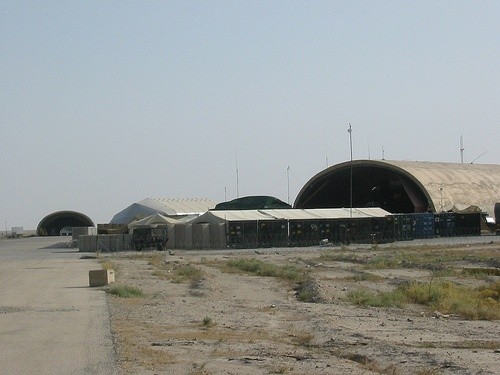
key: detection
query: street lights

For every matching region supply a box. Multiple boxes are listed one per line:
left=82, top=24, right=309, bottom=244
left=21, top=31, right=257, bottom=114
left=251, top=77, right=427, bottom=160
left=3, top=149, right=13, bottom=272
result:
left=347, top=123, right=354, bottom=218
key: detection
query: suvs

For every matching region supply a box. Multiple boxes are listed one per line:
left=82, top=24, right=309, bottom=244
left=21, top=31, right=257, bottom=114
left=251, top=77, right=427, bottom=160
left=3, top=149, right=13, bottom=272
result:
left=132, top=228, right=168, bottom=251
